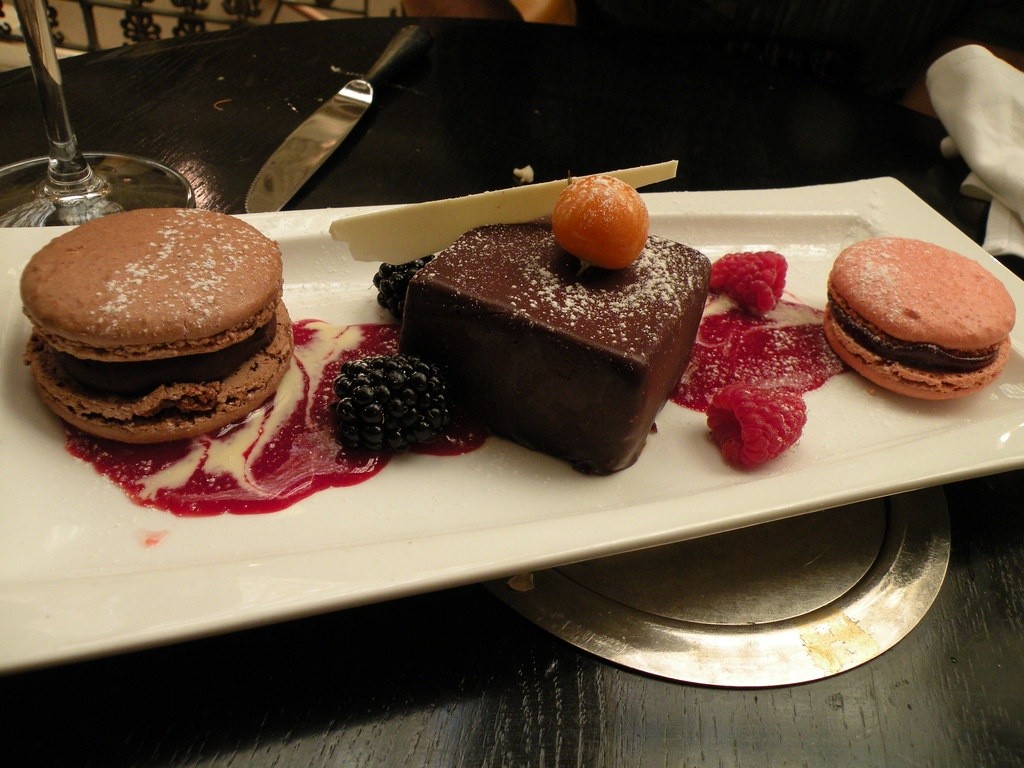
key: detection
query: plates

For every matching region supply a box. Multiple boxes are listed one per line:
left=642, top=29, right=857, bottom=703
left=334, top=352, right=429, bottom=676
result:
left=0, top=176, right=1024, bottom=677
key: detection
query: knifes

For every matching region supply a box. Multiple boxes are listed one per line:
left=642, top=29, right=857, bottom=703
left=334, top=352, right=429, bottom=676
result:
left=241, top=20, right=441, bottom=215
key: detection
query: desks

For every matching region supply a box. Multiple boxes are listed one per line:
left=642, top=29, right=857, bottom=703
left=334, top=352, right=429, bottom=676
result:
left=0, top=21, right=1024, bottom=767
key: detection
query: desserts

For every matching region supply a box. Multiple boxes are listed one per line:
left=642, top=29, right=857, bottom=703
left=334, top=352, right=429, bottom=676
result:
left=326, top=160, right=715, bottom=474
left=19, top=202, right=297, bottom=442
left=822, top=236, right=1016, bottom=398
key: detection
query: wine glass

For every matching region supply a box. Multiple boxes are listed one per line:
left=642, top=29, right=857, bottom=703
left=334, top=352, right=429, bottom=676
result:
left=0, top=0, right=197, bottom=229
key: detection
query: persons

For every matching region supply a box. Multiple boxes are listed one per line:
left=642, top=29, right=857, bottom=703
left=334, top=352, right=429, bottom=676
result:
left=404, top=1, right=1023, bottom=120
